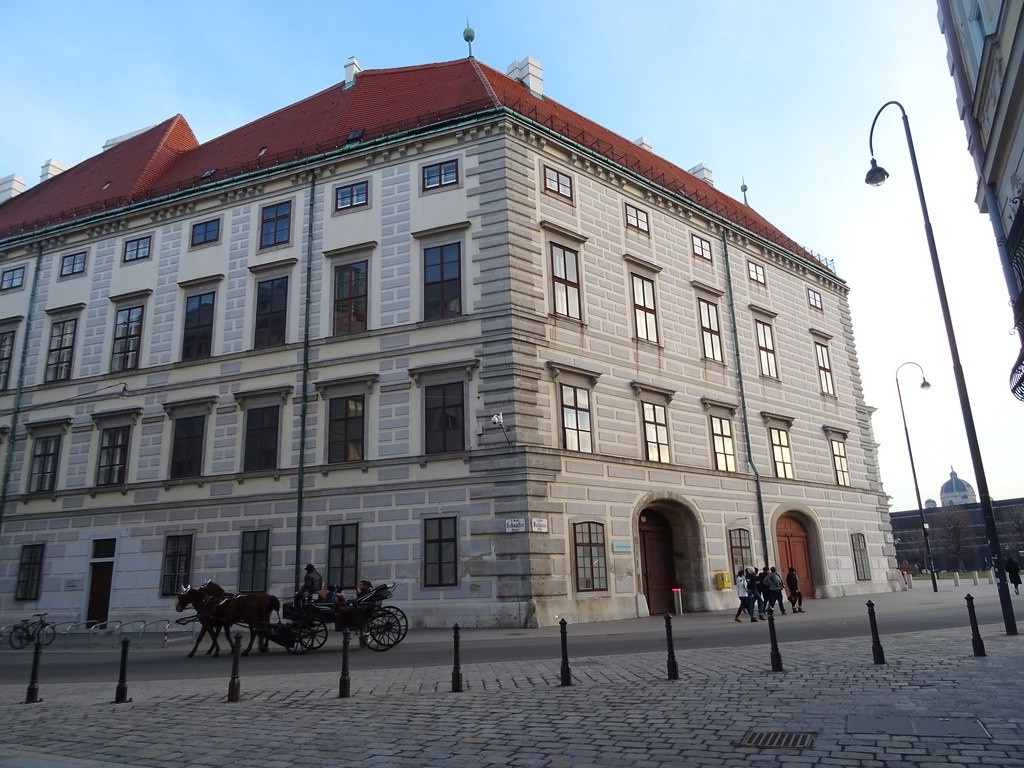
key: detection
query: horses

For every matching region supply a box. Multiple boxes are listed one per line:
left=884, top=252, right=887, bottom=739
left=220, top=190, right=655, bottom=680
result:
left=175, top=583, right=271, bottom=659
left=198, top=578, right=281, bottom=656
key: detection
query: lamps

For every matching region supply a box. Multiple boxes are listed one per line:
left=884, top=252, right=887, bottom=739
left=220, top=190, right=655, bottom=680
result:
left=490, top=411, right=511, bottom=447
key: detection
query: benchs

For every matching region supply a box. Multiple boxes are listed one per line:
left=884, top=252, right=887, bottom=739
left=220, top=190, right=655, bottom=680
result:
left=333, top=581, right=397, bottom=631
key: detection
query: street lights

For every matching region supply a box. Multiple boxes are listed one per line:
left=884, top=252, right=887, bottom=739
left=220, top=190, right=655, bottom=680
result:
left=896, top=362, right=942, bottom=593
left=865, top=101, right=1018, bottom=636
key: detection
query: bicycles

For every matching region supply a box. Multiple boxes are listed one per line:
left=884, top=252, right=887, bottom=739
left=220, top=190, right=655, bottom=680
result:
left=9, top=612, right=57, bottom=650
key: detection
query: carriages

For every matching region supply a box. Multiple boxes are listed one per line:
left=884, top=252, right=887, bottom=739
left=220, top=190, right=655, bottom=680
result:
left=173, top=563, right=409, bottom=655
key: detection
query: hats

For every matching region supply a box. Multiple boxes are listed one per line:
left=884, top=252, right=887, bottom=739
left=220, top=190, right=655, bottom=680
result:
left=304, top=564, right=314, bottom=571
left=747, top=566, right=756, bottom=574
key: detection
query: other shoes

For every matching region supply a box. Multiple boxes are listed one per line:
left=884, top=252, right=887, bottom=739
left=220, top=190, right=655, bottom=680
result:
left=782, top=611, right=787, bottom=615
left=735, top=617, right=741, bottom=622
left=759, top=615, right=765, bottom=620
left=798, top=606, right=805, bottom=612
left=751, top=617, right=758, bottom=621
left=793, top=608, right=799, bottom=613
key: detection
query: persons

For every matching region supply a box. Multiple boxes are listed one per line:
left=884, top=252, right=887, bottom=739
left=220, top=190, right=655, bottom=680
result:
left=295, top=564, right=373, bottom=612
left=1006, top=556, right=1022, bottom=594
left=734, top=566, right=806, bottom=622
left=915, top=563, right=923, bottom=575
